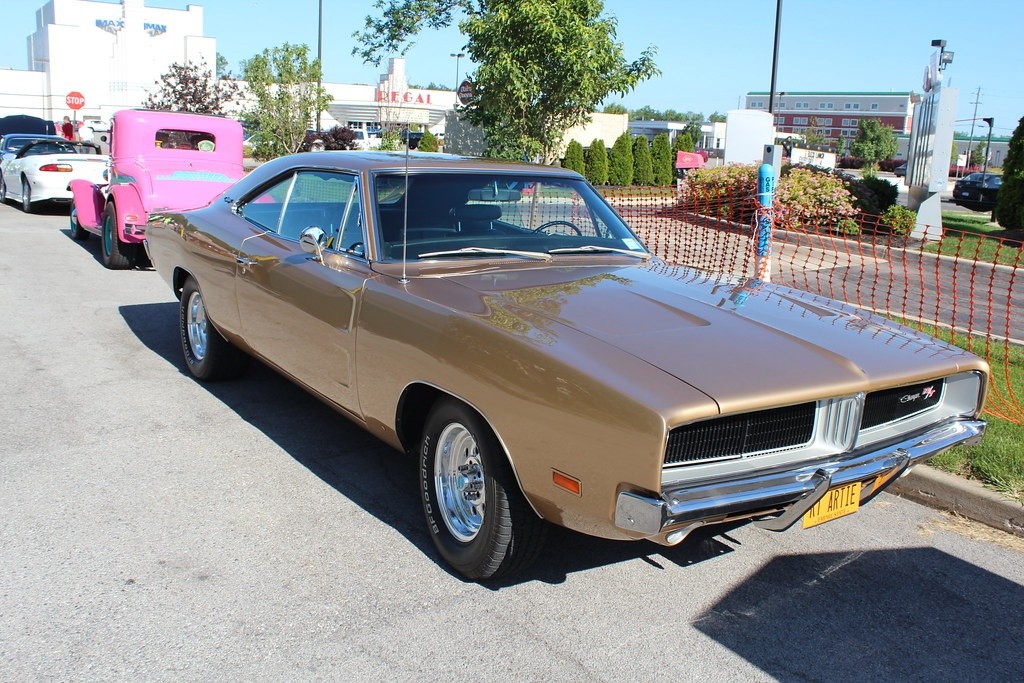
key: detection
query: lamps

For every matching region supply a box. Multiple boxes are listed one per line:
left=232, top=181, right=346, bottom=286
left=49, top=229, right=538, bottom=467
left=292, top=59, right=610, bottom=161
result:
left=931, top=39, right=954, bottom=70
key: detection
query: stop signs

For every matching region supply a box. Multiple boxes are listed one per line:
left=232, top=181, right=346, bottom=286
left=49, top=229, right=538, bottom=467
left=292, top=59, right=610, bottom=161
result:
left=66, top=92, right=85, bottom=109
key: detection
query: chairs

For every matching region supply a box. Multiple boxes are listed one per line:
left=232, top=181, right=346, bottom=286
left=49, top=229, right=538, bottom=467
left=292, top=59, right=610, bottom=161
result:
left=450, top=203, right=503, bottom=237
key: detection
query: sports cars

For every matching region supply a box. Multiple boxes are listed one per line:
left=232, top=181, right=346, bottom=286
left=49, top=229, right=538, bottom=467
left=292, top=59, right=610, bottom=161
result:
left=0, top=140, right=111, bottom=213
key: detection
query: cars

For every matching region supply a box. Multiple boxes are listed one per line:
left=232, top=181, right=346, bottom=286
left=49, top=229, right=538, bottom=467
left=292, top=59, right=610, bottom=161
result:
left=0, top=134, right=77, bottom=164
left=142, top=149, right=991, bottom=581
left=953, top=173, right=1003, bottom=203
left=55, top=119, right=111, bottom=132
left=67, top=108, right=276, bottom=271
left=894, top=163, right=907, bottom=177
left=695, top=146, right=708, bottom=163
left=242, top=128, right=445, bottom=150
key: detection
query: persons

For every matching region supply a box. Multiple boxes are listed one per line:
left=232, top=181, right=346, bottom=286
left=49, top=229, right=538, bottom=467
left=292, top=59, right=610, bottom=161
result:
left=77, top=122, right=94, bottom=143
left=62, top=115, right=73, bottom=140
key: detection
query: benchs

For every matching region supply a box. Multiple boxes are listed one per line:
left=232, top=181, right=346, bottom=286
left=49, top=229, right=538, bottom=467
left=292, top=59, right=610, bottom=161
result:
left=259, top=207, right=410, bottom=249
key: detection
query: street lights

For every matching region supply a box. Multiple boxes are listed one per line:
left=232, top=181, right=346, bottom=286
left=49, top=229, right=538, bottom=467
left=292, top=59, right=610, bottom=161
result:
left=931, top=39, right=947, bottom=72
left=450, top=53, right=465, bottom=103
left=776, top=92, right=785, bottom=145
